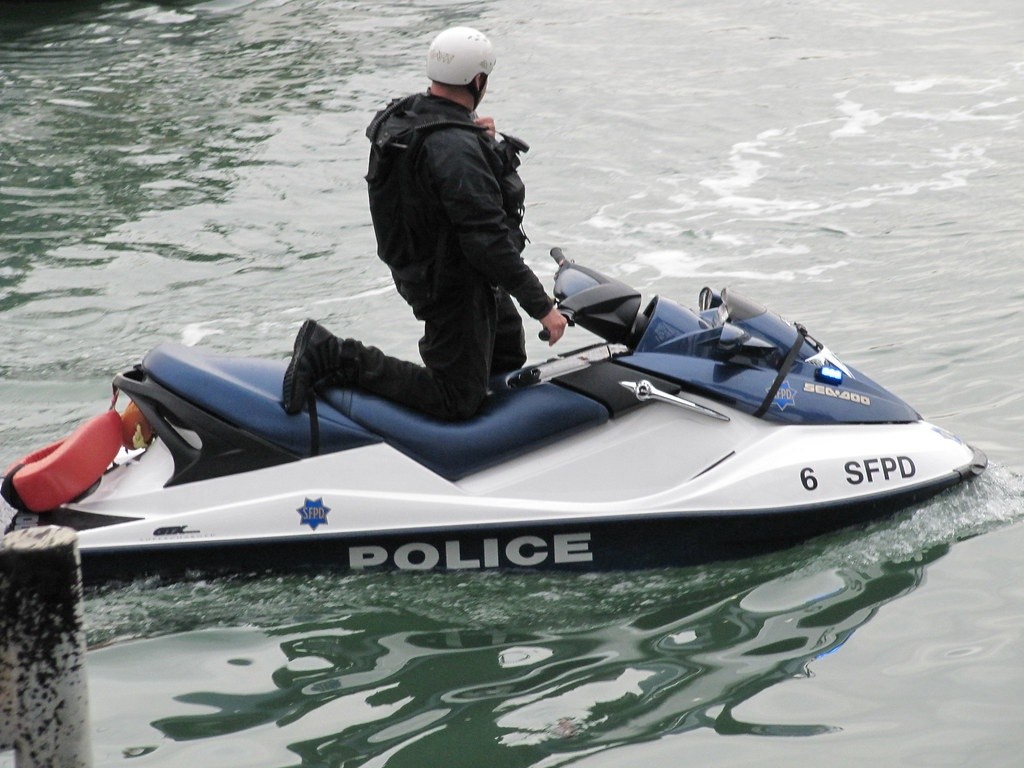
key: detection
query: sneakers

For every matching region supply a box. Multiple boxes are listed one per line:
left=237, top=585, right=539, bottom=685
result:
left=283, top=319, right=353, bottom=415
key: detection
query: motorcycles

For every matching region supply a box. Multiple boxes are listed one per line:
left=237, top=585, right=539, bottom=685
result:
left=0, top=245, right=990, bottom=584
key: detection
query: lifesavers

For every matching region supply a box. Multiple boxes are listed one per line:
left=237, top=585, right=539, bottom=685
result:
left=1, top=410, right=123, bottom=513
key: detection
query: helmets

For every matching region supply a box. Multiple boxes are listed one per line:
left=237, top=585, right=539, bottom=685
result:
left=425, top=26, right=496, bottom=86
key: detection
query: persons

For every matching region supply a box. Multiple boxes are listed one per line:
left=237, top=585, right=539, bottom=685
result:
left=283, top=23, right=568, bottom=422
